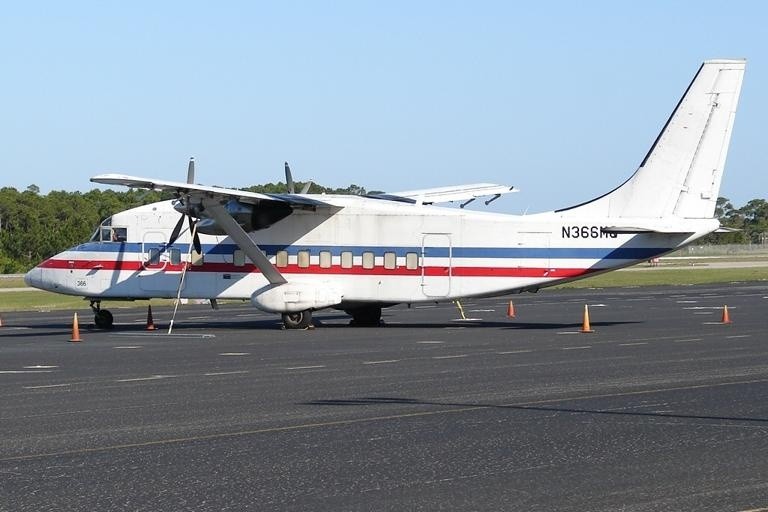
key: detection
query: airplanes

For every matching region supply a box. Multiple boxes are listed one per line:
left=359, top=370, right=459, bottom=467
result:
left=22, top=59, right=747, bottom=329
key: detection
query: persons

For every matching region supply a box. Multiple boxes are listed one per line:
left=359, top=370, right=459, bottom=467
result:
left=108, top=229, right=127, bottom=242
left=648, top=257, right=659, bottom=268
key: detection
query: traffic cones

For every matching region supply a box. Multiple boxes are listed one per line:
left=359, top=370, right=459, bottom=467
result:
left=64, top=312, right=83, bottom=343
left=578, top=303, right=596, bottom=332
left=142, top=304, right=159, bottom=331
left=505, top=298, right=516, bottom=319
left=719, top=304, right=735, bottom=323
